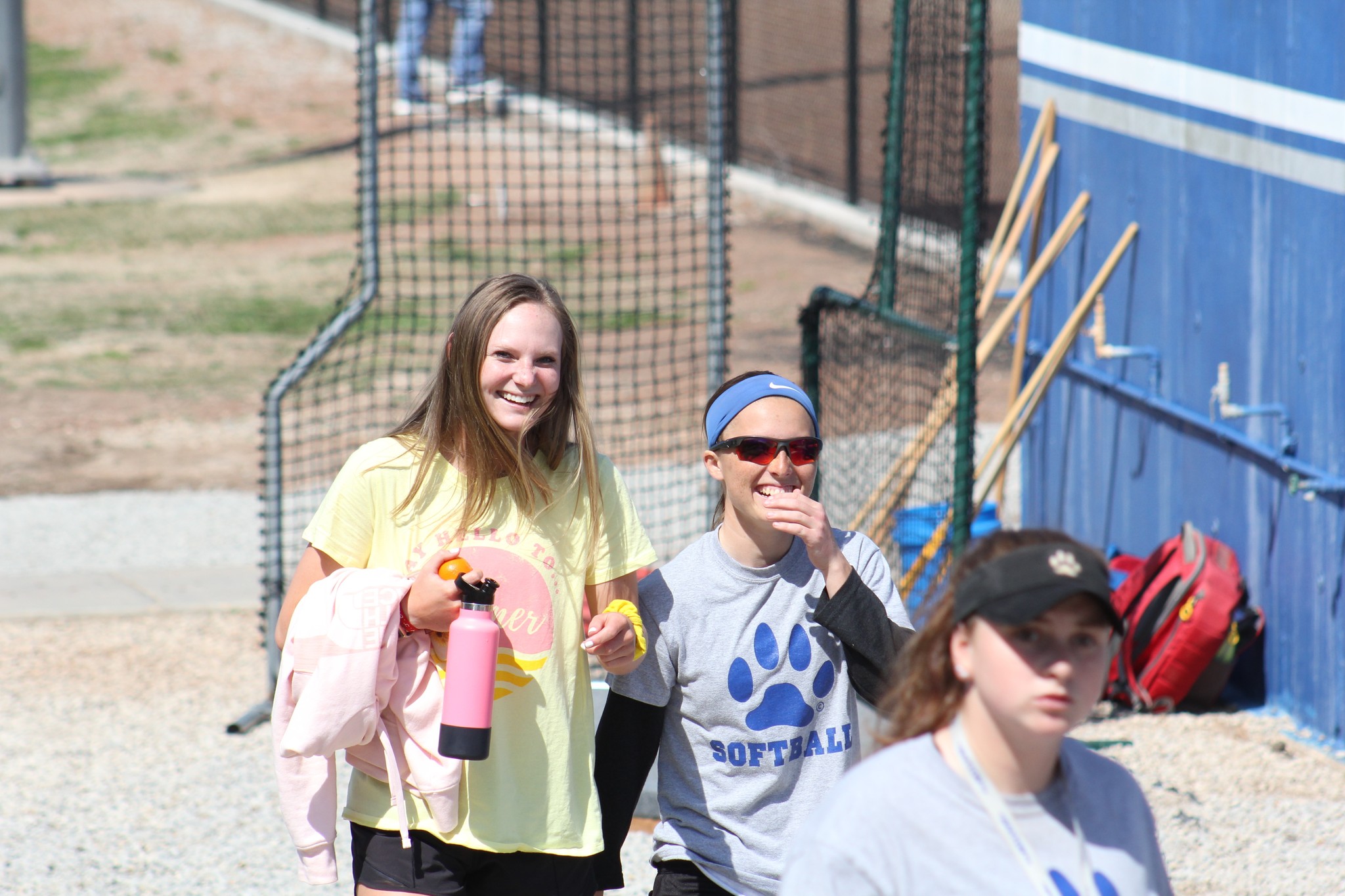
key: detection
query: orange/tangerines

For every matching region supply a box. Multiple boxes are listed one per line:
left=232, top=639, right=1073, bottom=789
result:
left=438, top=556, right=473, bottom=580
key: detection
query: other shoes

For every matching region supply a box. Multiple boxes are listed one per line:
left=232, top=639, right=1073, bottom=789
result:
left=454, top=79, right=503, bottom=93
left=397, top=100, right=445, bottom=114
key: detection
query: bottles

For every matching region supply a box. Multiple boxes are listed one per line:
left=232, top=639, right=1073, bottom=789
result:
left=437, top=572, right=499, bottom=760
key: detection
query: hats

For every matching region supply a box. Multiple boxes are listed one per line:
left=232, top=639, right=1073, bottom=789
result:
left=951, top=542, right=1125, bottom=637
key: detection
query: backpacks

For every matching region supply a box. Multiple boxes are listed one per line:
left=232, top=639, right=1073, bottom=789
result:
left=1104, top=521, right=1265, bottom=715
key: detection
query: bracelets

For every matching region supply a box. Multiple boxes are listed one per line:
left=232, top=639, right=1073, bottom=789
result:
left=601, top=600, right=647, bottom=661
left=400, top=602, right=419, bottom=632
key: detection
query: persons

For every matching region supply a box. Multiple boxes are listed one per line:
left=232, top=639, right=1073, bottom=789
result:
left=274, top=273, right=658, bottom=896
left=774, top=529, right=1176, bottom=895
left=593, top=370, right=917, bottom=896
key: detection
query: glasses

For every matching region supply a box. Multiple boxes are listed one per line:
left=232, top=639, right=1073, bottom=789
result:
left=710, top=436, right=823, bottom=466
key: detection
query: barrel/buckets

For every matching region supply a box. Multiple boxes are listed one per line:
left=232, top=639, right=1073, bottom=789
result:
left=894, top=502, right=999, bottom=636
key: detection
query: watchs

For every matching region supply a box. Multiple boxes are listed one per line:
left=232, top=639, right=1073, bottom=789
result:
left=398, top=624, right=413, bottom=640
